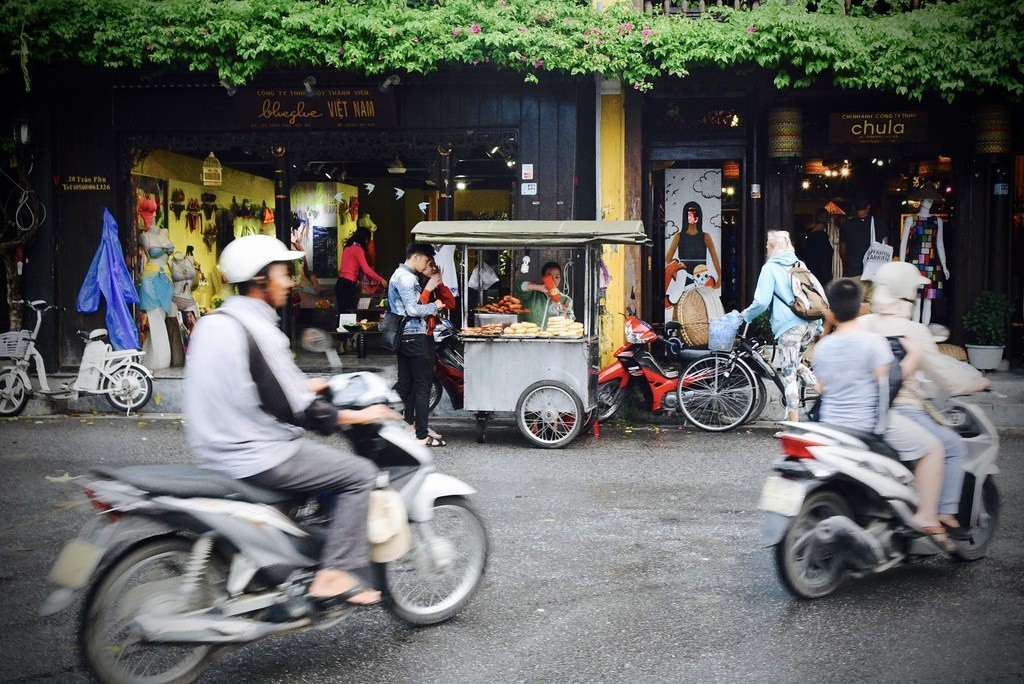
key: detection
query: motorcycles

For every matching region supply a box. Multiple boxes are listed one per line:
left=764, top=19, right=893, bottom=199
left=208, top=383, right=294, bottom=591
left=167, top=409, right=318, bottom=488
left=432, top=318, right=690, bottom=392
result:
left=596, top=307, right=767, bottom=432
left=39, top=332, right=489, bottom=684
left=409, top=220, right=651, bottom=450
left=753, top=324, right=1001, bottom=602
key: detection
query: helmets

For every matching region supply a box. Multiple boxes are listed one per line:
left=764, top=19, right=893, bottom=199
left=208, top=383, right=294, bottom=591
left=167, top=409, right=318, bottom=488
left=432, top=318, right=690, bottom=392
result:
left=433, top=318, right=458, bottom=343
left=871, top=261, right=932, bottom=300
left=217, top=233, right=306, bottom=283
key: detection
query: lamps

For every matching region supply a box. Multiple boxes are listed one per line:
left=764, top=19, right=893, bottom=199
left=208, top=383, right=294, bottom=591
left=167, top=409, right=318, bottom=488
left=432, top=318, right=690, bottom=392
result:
left=303, top=163, right=346, bottom=182
left=387, top=154, right=407, bottom=173
left=380, top=74, right=401, bottom=89
left=218, top=78, right=238, bottom=96
left=303, top=76, right=316, bottom=92
left=485, top=144, right=516, bottom=169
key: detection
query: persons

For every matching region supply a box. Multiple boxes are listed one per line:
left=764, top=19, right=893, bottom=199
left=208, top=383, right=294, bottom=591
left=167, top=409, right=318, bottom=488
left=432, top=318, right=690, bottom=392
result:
left=337, top=227, right=388, bottom=312
left=138, top=226, right=198, bottom=315
left=385, top=242, right=576, bottom=448
left=665, top=201, right=722, bottom=290
left=809, top=195, right=989, bottom=554
left=186, top=235, right=405, bottom=613
left=899, top=184, right=949, bottom=326
left=741, top=229, right=831, bottom=439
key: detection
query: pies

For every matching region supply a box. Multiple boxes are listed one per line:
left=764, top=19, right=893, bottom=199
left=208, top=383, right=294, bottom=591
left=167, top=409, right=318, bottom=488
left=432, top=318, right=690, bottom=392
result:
left=461, top=322, right=508, bottom=335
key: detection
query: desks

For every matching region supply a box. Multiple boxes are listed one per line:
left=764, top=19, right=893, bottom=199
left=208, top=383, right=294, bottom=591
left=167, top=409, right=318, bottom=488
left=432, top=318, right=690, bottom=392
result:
left=329, top=331, right=385, bottom=359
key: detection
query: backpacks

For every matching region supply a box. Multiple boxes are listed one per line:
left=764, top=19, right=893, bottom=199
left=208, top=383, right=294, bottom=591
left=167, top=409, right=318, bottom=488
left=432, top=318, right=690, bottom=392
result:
left=774, top=260, right=829, bottom=321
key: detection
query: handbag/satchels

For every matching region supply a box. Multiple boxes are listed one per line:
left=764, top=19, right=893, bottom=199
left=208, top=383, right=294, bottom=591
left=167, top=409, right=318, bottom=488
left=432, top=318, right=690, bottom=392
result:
left=859, top=215, right=894, bottom=282
left=378, top=312, right=412, bottom=353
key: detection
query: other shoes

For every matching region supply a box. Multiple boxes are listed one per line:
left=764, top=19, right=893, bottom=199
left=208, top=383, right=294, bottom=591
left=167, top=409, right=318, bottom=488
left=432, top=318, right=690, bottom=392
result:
left=939, top=519, right=972, bottom=539
left=773, top=431, right=783, bottom=438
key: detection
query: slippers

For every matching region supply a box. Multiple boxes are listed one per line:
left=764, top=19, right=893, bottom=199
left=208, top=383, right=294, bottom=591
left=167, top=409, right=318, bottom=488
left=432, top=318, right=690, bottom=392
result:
left=304, top=580, right=387, bottom=609
left=408, top=424, right=447, bottom=447
left=906, top=520, right=957, bottom=552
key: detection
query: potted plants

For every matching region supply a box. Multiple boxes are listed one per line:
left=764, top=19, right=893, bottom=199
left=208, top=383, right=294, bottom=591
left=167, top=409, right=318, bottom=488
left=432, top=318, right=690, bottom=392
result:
left=962, top=292, right=1016, bottom=369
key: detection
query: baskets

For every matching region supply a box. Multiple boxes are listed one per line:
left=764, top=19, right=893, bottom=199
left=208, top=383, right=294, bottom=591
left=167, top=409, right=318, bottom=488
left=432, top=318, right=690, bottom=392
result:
left=0, top=330, right=32, bottom=358
left=708, top=316, right=744, bottom=352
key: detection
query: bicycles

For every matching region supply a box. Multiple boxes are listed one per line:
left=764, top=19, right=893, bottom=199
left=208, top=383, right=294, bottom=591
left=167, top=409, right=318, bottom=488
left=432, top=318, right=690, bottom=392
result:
left=676, top=314, right=891, bottom=433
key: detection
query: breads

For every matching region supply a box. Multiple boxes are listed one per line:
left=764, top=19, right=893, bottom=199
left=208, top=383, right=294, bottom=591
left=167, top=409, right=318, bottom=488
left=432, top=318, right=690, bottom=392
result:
left=477, top=295, right=532, bottom=314
left=503, top=315, right=584, bottom=338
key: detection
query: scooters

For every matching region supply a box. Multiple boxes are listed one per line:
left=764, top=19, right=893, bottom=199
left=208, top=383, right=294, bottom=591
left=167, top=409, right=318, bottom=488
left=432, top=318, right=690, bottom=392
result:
left=0, top=297, right=156, bottom=416
